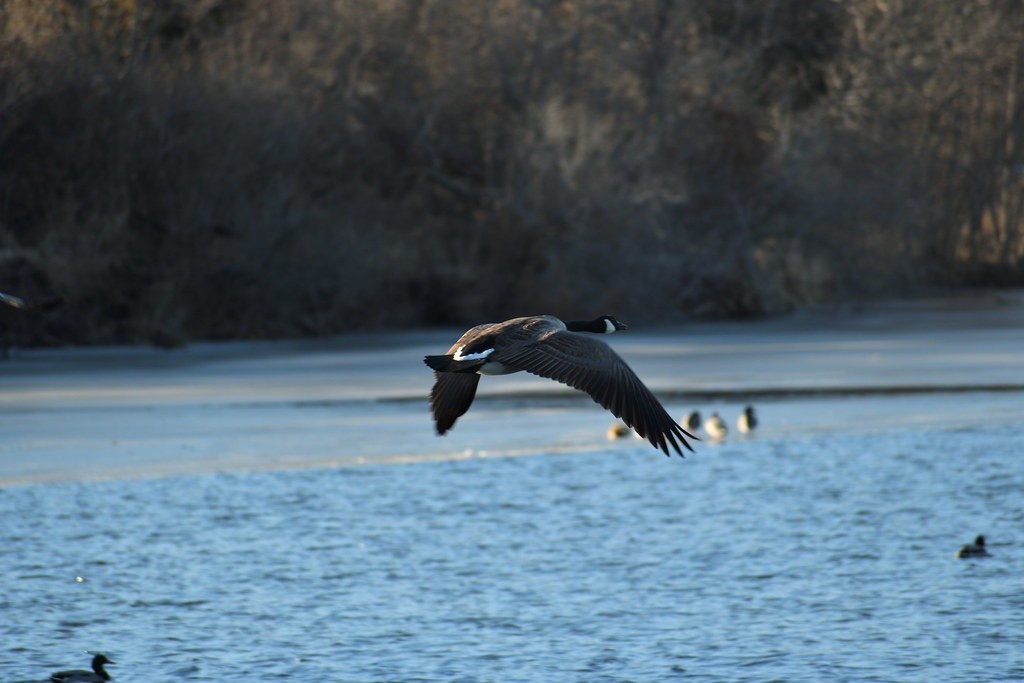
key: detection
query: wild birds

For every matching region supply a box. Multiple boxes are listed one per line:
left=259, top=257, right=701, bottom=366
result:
left=958, top=532, right=994, bottom=561
left=679, top=410, right=702, bottom=433
left=704, top=412, right=729, bottom=438
left=606, top=423, right=631, bottom=442
left=735, top=404, right=760, bottom=433
left=46, top=653, right=117, bottom=683
left=422, top=314, right=702, bottom=457
